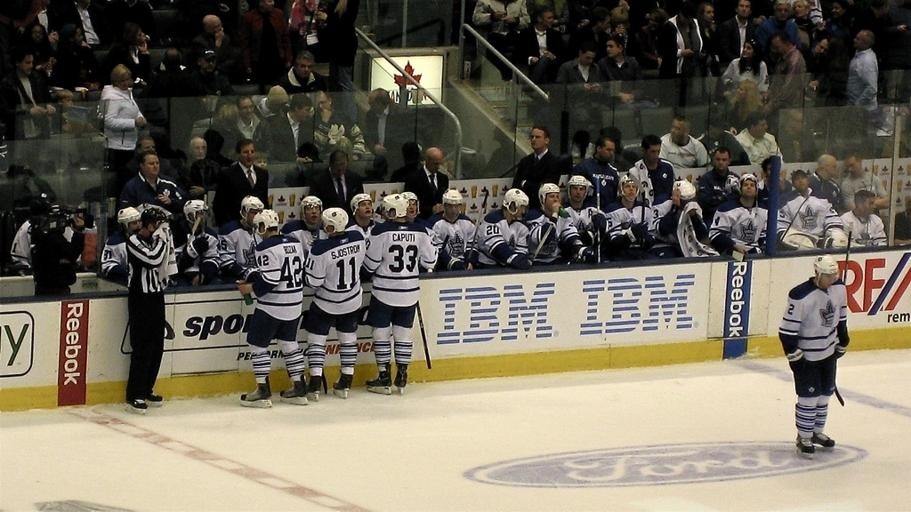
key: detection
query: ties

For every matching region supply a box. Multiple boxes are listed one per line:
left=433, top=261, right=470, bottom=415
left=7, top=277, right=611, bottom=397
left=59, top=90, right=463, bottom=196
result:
left=336, top=177, right=345, bottom=201
left=247, top=170, right=254, bottom=189
left=429, top=173, right=435, bottom=184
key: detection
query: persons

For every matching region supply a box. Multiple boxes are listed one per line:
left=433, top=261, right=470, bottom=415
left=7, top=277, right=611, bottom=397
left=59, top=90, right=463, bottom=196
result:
left=0, top=164, right=56, bottom=221
left=119, top=153, right=186, bottom=246
left=476, top=188, right=532, bottom=270
left=30, top=219, right=86, bottom=296
left=573, top=133, right=627, bottom=203
left=125, top=209, right=179, bottom=408
left=659, top=115, right=710, bottom=167
left=780, top=255, right=850, bottom=460
left=893, top=191, right=911, bottom=246
left=607, top=172, right=659, bottom=260
left=806, top=154, right=842, bottom=214
left=172, top=199, right=220, bottom=284
left=213, top=140, right=270, bottom=236
left=237, top=209, right=308, bottom=406
left=472, top=1, right=699, bottom=128
left=431, top=189, right=479, bottom=272
left=777, top=112, right=819, bottom=161
left=363, top=89, right=412, bottom=157
left=841, top=153, right=889, bottom=212
left=9, top=195, right=86, bottom=276
left=700, top=110, right=751, bottom=166
left=279, top=196, right=329, bottom=257
left=178, top=137, right=223, bottom=203
left=362, top=192, right=437, bottom=394
left=309, top=151, right=363, bottom=212
left=304, top=206, right=366, bottom=401
left=555, top=173, right=611, bottom=264
left=345, top=192, right=382, bottom=248
left=401, top=192, right=433, bottom=236
left=0, top=0, right=168, bottom=175
left=512, top=125, right=568, bottom=207
left=650, top=179, right=708, bottom=257
left=528, top=181, right=573, bottom=267
left=708, top=173, right=769, bottom=255
left=699, top=1, right=910, bottom=131
left=630, top=135, right=674, bottom=205
left=735, top=115, right=785, bottom=167
left=756, top=158, right=790, bottom=205
left=168, top=1, right=365, bottom=154
left=220, top=194, right=269, bottom=284
left=99, top=205, right=145, bottom=286
left=776, top=170, right=844, bottom=252
left=841, top=188, right=887, bottom=249
left=409, top=145, right=449, bottom=214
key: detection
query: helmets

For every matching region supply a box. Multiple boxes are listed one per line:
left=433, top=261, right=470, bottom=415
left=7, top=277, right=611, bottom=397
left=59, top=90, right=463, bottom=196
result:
left=740, top=174, right=758, bottom=192
left=814, top=255, right=838, bottom=275
left=117, top=207, right=141, bottom=229
left=617, top=174, right=640, bottom=197
left=538, top=183, right=560, bottom=204
left=401, top=192, right=421, bottom=215
left=568, top=176, right=588, bottom=200
left=350, top=194, right=371, bottom=216
left=502, top=188, right=529, bottom=215
left=183, top=200, right=209, bottom=224
left=383, top=194, right=409, bottom=219
left=240, top=195, right=264, bottom=219
left=253, top=209, right=279, bottom=235
left=141, top=208, right=166, bottom=226
left=300, top=196, right=323, bottom=218
left=443, top=190, right=463, bottom=205
left=321, top=208, right=349, bottom=234
left=673, top=179, right=696, bottom=200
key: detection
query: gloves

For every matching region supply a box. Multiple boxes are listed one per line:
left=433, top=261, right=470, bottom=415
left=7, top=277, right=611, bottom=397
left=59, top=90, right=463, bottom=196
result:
left=787, top=349, right=807, bottom=374
left=832, top=345, right=846, bottom=359
left=725, top=174, right=740, bottom=192
left=578, top=246, right=594, bottom=262
left=193, top=236, right=209, bottom=254
left=581, top=230, right=597, bottom=246
left=627, top=221, right=649, bottom=243
left=507, top=253, right=533, bottom=269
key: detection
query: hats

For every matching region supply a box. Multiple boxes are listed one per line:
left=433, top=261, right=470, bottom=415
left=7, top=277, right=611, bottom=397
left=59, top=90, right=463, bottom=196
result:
left=201, top=48, right=216, bottom=59
left=268, top=86, right=288, bottom=104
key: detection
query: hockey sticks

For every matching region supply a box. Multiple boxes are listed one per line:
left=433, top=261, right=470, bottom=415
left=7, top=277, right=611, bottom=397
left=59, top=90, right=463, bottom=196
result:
left=779, top=187, right=813, bottom=244
left=835, top=231, right=852, bottom=406
left=627, top=173, right=646, bottom=259
left=467, top=186, right=488, bottom=269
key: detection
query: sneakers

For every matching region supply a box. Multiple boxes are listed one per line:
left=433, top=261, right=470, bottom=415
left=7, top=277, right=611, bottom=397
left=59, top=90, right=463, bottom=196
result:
left=306, top=376, right=321, bottom=392
left=394, top=370, right=407, bottom=387
left=130, top=398, right=147, bottom=409
left=241, top=383, right=270, bottom=401
left=333, top=373, right=352, bottom=389
left=812, top=431, right=835, bottom=446
left=367, top=372, right=391, bottom=386
left=280, top=381, right=305, bottom=398
left=148, top=394, right=162, bottom=401
left=796, top=436, right=814, bottom=454
